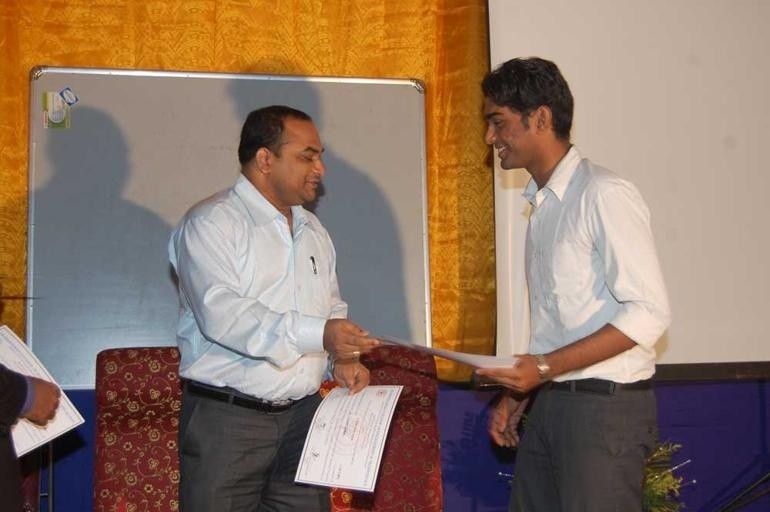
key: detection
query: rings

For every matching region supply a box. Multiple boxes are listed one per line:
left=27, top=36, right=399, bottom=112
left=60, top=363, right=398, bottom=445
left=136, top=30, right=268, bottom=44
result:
left=347, top=380, right=355, bottom=385
left=352, top=350, right=362, bottom=358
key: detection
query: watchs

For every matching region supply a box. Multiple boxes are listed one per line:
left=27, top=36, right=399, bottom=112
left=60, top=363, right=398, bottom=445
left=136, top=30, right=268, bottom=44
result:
left=535, top=353, right=550, bottom=385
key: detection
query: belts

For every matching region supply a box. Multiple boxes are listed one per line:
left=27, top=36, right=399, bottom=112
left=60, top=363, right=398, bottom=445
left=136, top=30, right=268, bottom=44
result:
left=180, top=381, right=307, bottom=413
left=539, top=376, right=656, bottom=397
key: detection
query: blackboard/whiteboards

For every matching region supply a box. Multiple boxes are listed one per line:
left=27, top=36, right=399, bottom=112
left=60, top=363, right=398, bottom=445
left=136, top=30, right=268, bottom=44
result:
left=24, top=66, right=433, bottom=388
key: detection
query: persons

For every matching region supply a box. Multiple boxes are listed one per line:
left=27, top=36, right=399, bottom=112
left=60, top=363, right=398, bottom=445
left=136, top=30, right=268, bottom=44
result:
left=0, top=362, right=60, bottom=510
left=480, top=56, right=671, bottom=510
left=166, top=106, right=378, bottom=512
